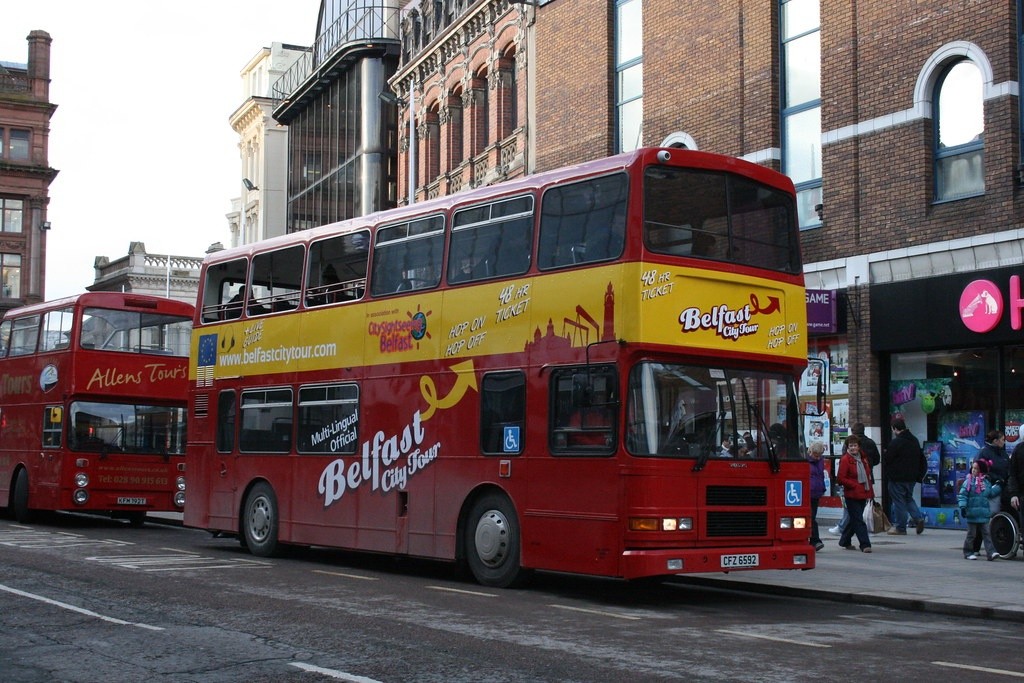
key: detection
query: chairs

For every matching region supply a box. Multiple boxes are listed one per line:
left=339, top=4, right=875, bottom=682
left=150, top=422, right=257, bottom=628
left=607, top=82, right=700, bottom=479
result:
left=396, top=279, right=412, bottom=291
left=355, top=282, right=366, bottom=298
left=484, top=256, right=491, bottom=277
left=271, top=297, right=290, bottom=312
left=568, top=243, right=585, bottom=263
left=324, top=288, right=338, bottom=303
left=219, top=303, right=228, bottom=320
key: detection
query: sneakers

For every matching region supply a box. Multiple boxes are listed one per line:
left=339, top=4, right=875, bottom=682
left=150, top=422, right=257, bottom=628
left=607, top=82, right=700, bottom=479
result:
left=829, top=525, right=841, bottom=536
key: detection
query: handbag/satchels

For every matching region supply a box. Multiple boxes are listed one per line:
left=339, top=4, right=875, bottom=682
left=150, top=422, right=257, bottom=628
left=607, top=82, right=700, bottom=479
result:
left=916, top=452, right=928, bottom=483
left=862, top=498, right=891, bottom=533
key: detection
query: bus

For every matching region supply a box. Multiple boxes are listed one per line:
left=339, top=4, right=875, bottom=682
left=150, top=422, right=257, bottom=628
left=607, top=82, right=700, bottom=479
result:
left=182, top=144, right=827, bottom=591
left=0, top=290, right=196, bottom=525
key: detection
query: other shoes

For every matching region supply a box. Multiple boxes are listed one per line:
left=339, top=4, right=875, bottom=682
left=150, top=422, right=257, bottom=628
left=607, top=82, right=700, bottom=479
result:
left=840, top=542, right=855, bottom=550
left=815, top=543, right=825, bottom=550
left=966, top=554, right=978, bottom=560
left=988, top=553, right=998, bottom=561
left=863, top=546, right=873, bottom=553
left=915, top=518, right=924, bottom=534
left=888, top=529, right=907, bottom=535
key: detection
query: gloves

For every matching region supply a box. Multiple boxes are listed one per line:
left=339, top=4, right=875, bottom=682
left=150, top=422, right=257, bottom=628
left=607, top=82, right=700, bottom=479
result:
left=960, top=508, right=966, bottom=517
left=996, top=479, right=1003, bottom=488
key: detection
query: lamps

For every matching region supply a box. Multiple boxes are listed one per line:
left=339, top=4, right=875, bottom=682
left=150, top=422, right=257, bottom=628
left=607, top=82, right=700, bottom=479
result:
left=243, top=177, right=257, bottom=191
left=40, top=221, right=52, bottom=231
left=377, top=91, right=408, bottom=109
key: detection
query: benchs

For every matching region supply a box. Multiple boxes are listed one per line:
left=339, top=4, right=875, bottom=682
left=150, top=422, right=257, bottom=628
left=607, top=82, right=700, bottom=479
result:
left=132, top=345, right=174, bottom=355
left=118, top=346, right=134, bottom=352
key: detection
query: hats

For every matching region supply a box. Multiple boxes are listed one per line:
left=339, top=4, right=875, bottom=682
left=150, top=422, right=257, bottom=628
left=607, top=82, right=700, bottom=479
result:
left=851, top=422, right=865, bottom=435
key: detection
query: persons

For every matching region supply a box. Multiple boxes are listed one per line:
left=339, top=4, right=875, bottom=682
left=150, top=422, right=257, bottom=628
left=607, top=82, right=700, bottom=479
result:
left=829, top=423, right=881, bottom=535
left=973, top=431, right=1020, bottom=529
left=318, top=261, right=347, bottom=303
left=569, top=384, right=634, bottom=446
left=468, top=237, right=530, bottom=279
left=226, top=286, right=267, bottom=319
left=882, top=418, right=924, bottom=534
left=1009, top=425, right=1024, bottom=554
left=958, top=458, right=1002, bottom=560
left=727, top=246, right=740, bottom=260
left=582, top=206, right=626, bottom=262
left=486, top=392, right=523, bottom=453
left=691, top=235, right=716, bottom=257
left=807, top=442, right=825, bottom=551
left=720, top=423, right=786, bottom=459
left=837, top=435, right=875, bottom=553
